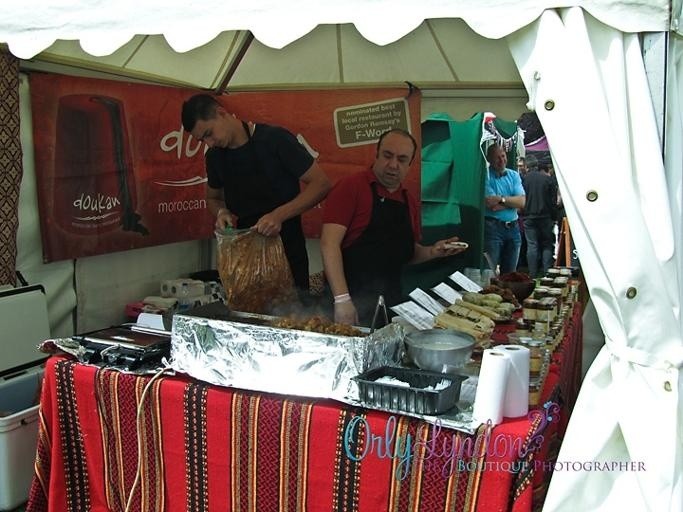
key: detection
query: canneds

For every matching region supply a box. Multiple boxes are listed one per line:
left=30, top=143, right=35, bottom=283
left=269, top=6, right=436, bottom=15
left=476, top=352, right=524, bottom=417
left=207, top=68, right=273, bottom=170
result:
left=515, top=268, right=578, bottom=410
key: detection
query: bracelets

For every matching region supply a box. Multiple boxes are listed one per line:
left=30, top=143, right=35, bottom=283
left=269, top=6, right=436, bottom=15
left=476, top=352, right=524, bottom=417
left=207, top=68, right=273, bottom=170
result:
left=499, top=197, right=506, bottom=205
left=333, top=293, right=351, bottom=304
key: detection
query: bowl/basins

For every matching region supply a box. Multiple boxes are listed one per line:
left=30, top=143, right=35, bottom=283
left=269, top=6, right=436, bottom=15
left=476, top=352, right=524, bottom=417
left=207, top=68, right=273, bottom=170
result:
left=403, top=329, right=476, bottom=374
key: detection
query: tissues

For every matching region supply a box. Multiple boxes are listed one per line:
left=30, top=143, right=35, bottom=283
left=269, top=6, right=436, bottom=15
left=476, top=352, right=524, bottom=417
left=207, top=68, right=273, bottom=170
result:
left=160, top=276, right=218, bottom=309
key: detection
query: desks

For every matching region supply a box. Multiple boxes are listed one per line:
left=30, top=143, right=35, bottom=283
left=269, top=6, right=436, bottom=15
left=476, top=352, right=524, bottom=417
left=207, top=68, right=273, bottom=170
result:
left=25, top=302, right=584, bottom=512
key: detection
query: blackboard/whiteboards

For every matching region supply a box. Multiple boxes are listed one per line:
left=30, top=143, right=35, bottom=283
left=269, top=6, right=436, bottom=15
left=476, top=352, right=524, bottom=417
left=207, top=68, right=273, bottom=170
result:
left=565, top=218, right=581, bottom=284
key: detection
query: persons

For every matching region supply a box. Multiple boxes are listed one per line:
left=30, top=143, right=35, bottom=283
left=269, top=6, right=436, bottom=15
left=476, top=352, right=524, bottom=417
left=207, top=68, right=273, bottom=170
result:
left=484, top=143, right=527, bottom=277
left=317, top=128, right=467, bottom=325
left=517, top=155, right=580, bottom=277
left=181, top=93, right=331, bottom=294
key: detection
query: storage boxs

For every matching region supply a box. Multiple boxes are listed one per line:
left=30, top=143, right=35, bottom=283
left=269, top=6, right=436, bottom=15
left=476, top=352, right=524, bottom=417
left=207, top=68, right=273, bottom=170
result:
left=0, top=271, right=52, bottom=512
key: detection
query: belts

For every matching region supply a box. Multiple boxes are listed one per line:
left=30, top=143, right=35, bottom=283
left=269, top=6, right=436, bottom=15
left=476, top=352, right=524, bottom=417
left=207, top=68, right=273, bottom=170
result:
left=486, top=217, right=519, bottom=229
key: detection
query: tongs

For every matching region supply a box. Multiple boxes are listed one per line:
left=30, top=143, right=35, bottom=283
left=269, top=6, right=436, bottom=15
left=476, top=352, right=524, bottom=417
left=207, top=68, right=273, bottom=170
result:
left=369, top=295, right=390, bottom=335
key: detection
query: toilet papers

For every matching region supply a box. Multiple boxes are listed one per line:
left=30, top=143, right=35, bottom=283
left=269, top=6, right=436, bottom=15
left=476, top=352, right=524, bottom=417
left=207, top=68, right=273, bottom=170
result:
left=472, top=348, right=507, bottom=427
left=495, top=344, right=531, bottom=419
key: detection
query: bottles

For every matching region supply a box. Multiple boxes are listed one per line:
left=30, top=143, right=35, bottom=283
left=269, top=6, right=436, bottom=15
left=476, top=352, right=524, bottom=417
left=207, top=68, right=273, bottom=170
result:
left=505, top=329, right=549, bottom=410
